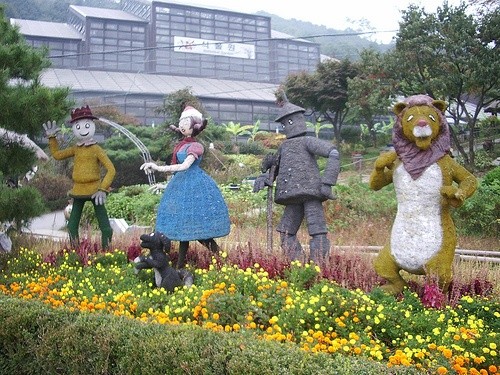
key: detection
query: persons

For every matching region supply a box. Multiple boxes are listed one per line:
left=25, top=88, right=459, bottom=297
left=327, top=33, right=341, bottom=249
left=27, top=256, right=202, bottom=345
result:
left=140, top=104, right=231, bottom=273
left=41, top=104, right=116, bottom=256
left=251, top=89, right=342, bottom=268
